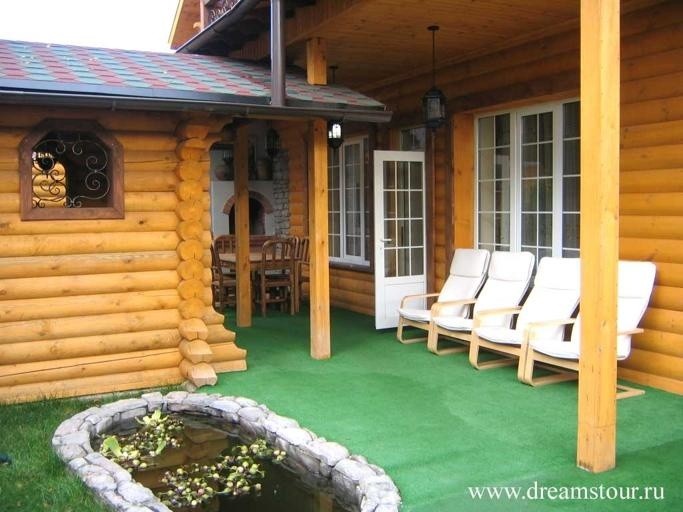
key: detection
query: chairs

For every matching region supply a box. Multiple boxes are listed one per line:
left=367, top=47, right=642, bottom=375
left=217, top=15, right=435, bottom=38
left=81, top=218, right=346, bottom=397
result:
left=473, top=255, right=584, bottom=387
left=524, top=258, right=658, bottom=401
left=209, top=233, right=309, bottom=320
left=432, top=249, right=536, bottom=372
left=389, top=246, right=490, bottom=359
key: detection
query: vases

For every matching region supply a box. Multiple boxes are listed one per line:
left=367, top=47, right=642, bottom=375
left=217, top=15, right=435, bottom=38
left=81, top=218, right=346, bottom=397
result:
left=256, top=162, right=272, bottom=180
left=214, top=165, right=233, bottom=181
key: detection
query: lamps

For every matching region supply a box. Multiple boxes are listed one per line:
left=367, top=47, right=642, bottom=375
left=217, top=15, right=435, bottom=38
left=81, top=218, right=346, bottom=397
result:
left=222, top=147, right=233, bottom=166
left=324, top=64, right=344, bottom=158
left=419, top=23, right=448, bottom=133
left=265, top=128, right=279, bottom=159
